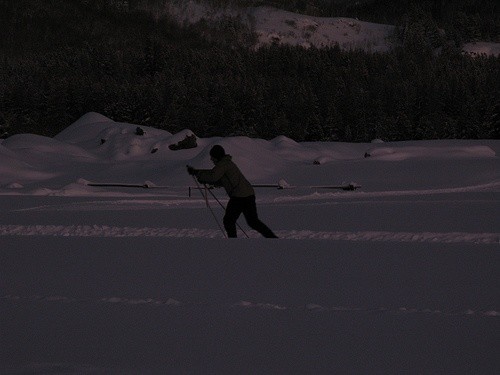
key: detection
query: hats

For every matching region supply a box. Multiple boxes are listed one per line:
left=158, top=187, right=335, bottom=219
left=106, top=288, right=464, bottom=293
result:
left=210, top=145, right=225, bottom=159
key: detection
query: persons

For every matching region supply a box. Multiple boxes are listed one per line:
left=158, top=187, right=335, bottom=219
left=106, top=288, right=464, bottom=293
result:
left=186, top=145, right=278, bottom=238
